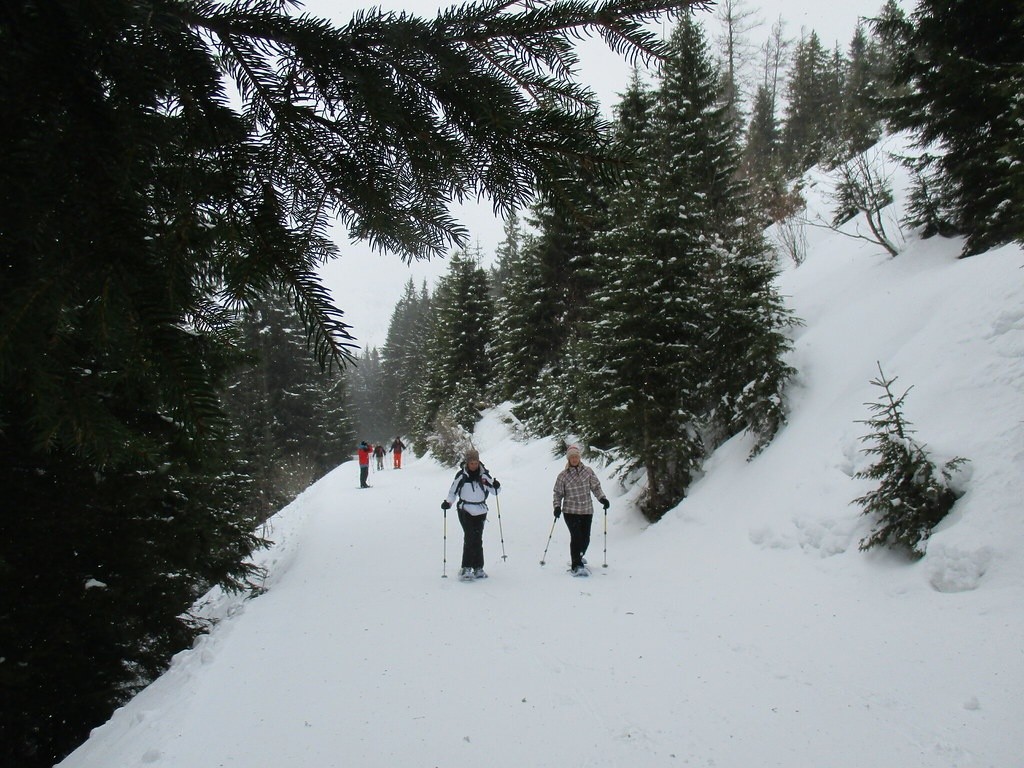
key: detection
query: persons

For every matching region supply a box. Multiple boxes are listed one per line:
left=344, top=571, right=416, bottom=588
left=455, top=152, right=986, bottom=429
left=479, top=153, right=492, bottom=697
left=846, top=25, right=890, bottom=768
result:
left=440, top=449, right=503, bottom=583
left=389, top=436, right=406, bottom=470
left=552, top=445, right=610, bottom=574
left=358, top=441, right=373, bottom=489
left=371, top=441, right=387, bottom=470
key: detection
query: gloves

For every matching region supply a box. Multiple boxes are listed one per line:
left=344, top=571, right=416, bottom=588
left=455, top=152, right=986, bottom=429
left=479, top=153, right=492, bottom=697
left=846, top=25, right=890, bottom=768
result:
left=493, top=478, right=500, bottom=488
left=600, top=498, right=609, bottom=509
left=441, top=500, right=450, bottom=509
left=554, top=507, right=561, bottom=518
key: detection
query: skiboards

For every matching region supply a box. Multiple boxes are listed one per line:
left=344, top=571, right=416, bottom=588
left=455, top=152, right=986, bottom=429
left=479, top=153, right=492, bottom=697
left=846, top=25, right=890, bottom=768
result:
left=458, top=569, right=490, bottom=583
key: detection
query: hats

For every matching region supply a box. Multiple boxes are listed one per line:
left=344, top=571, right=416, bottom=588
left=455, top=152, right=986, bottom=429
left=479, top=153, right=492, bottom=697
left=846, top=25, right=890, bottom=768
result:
left=465, top=450, right=479, bottom=463
left=361, top=441, right=367, bottom=446
left=376, top=441, right=380, bottom=444
left=566, top=445, right=580, bottom=459
left=396, top=436, right=400, bottom=440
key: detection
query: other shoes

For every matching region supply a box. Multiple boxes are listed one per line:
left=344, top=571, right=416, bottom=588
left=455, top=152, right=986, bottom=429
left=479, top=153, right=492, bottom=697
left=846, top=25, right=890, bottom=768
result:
left=581, top=558, right=587, bottom=564
left=462, top=567, right=472, bottom=578
left=475, top=568, right=486, bottom=578
left=571, top=566, right=584, bottom=574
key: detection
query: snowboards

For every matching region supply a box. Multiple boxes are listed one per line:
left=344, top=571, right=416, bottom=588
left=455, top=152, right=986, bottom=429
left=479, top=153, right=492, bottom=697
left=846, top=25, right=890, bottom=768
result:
left=572, top=568, right=589, bottom=577
